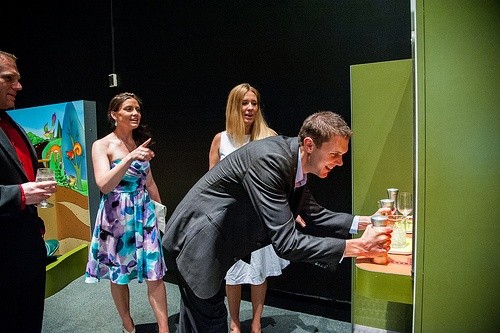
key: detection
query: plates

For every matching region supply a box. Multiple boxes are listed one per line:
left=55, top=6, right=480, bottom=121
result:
left=393, top=215, right=413, bottom=233
left=388, top=238, right=412, bottom=255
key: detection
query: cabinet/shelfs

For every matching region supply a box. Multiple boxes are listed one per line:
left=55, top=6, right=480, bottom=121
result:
left=349, top=1, right=500, bottom=333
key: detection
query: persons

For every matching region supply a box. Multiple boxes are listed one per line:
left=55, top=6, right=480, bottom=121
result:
left=208, top=82, right=291, bottom=333
left=0, top=50, right=57, bottom=333
left=161, top=112, right=395, bottom=333
left=84, top=92, right=170, bottom=333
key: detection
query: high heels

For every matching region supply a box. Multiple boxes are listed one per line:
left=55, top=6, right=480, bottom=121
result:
left=123, top=321, right=136, bottom=333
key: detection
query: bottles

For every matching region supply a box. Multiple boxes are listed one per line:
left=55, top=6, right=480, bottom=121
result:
left=386, top=187, right=404, bottom=215
left=378, top=198, right=394, bottom=225
left=370, top=215, right=389, bottom=264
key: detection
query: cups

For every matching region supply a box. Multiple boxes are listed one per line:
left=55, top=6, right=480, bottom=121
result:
left=388, top=215, right=406, bottom=247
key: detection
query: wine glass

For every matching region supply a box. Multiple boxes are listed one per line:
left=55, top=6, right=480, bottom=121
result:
left=34, top=169, right=55, bottom=209
left=396, top=192, right=413, bottom=220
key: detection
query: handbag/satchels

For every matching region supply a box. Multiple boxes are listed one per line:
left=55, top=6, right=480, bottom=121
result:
left=151, top=199, right=167, bottom=235
